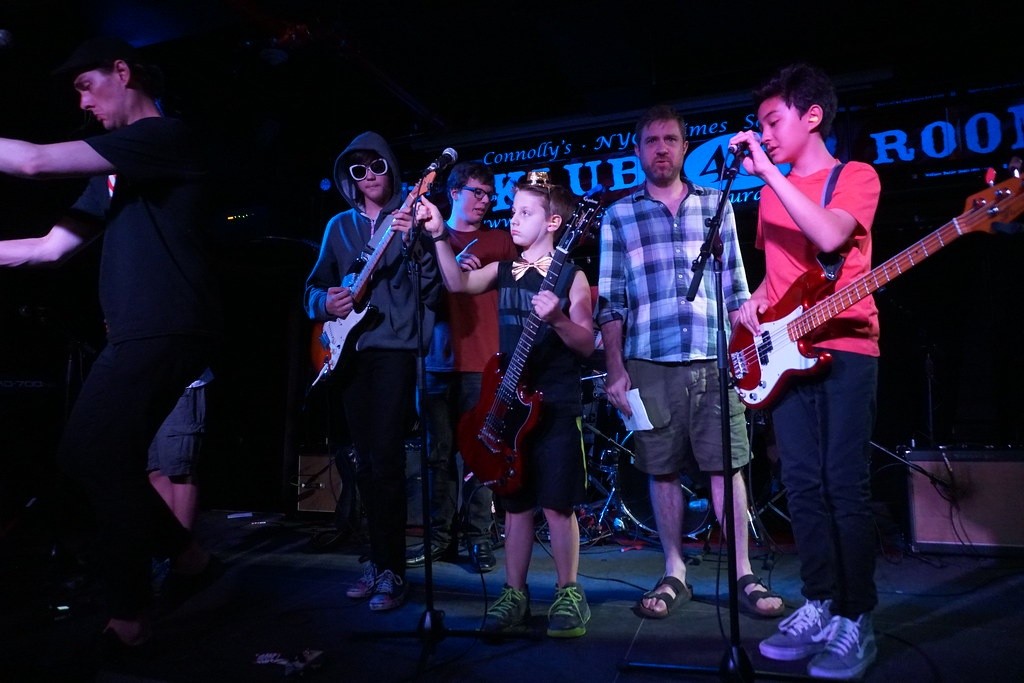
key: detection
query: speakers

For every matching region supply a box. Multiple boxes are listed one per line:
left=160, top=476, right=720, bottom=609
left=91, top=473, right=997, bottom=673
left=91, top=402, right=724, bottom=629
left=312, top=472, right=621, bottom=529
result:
left=905, top=448, right=1024, bottom=557
left=295, top=444, right=343, bottom=514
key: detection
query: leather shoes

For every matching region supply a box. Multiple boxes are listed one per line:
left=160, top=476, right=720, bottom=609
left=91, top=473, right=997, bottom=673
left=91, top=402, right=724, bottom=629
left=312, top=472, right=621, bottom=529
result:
left=469, top=543, right=497, bottom=572
left=405, top=544, right=456, bottom=567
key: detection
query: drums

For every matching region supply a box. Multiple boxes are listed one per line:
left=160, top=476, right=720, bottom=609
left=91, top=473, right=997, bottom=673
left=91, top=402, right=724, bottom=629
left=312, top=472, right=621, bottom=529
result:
left=614, top=429, right=718, bottom=540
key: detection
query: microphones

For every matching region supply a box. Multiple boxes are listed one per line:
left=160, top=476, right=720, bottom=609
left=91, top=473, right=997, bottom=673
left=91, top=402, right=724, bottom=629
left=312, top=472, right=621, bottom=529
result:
left=728, top=132, right=761, bottom=153
left=423, top=148, right=458, bottom=176
left=689, top=499, right=708, bottom=512
left=299, top=482, right=325, bottom=490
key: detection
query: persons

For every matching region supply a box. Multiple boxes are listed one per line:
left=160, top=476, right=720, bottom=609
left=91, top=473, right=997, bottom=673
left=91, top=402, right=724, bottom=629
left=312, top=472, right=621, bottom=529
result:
left=413, top=180, right=594, bottom=638
left=728, top=63, right=880, bottom=682
left=299, top=131, right=446, bottom=612
left=595, top=104, right=787, bottom=619
left=403, top=161, right=525, bottom=572
left=0, top=37, right=223, bottom=683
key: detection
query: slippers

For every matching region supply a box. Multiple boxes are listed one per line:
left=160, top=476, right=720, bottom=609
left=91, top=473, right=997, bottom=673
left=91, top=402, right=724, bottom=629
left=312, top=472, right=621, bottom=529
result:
left=737, top=575, right=785, bottom=618
left=638, top=576, right=693, bottom=619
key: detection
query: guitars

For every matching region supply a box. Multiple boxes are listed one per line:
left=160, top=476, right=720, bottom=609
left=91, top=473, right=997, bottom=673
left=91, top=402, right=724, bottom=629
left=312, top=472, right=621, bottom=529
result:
left=727, top=158, right=1024, bottom=409
left=309, top=171, right=437, bottom=381
left=456, top=192, right=606, bottom=494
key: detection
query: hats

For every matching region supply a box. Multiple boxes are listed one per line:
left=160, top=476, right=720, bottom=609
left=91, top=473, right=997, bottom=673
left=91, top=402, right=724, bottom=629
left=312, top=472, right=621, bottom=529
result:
left=62, top=36, right=142, bottom=83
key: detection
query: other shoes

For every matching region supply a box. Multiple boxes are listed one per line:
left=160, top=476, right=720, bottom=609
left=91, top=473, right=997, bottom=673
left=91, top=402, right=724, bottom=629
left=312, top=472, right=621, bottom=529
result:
left=70, top=628, right=159, bottom=670
left=146, top=569, right=184, bottom=616
left=181, top=554, right=225, bottom=595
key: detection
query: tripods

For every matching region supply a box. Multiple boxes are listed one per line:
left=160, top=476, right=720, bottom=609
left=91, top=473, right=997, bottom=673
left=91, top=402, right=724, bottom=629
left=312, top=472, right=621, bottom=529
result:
left=350, top=149, right=544, bottom=683
left=616, top=144, right=838, bottom=683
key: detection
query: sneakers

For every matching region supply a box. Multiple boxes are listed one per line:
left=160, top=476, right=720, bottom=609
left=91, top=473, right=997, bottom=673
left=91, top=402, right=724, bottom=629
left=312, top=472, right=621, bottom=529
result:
left=485, top=582, right=531, bottom=632
left=546, top=582, right=591, bottom=638
left=369, top=570, right=409, bottom=611
left=759, top=599, right=834, bottom=662
left=346, top=560, right=378, bottom=598
left=806, top=612, right=878, bottom=679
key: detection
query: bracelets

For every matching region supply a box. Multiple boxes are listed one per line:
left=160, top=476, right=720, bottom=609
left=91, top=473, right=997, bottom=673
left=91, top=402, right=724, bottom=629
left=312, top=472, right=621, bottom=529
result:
left=433, top=230, right=448, bottom=243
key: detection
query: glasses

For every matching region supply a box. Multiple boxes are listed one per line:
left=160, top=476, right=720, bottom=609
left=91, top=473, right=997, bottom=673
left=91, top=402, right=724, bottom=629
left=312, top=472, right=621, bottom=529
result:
left=459, top=185, right=498, bottom=203
left=349, top=158, right=388, bottom=181
